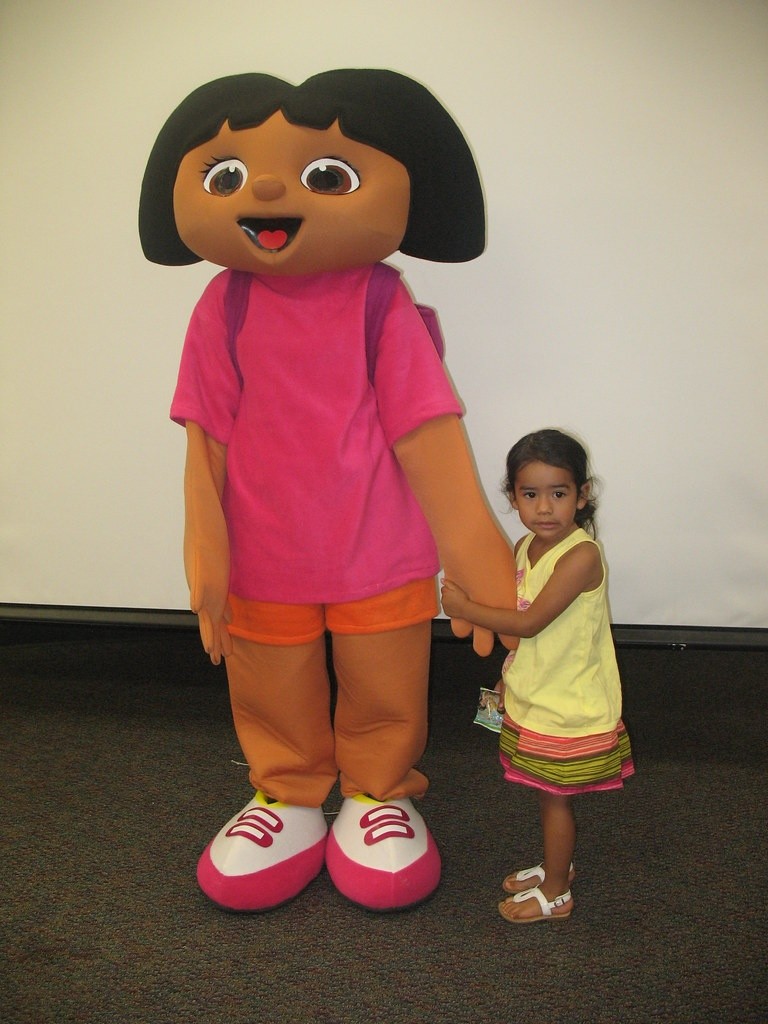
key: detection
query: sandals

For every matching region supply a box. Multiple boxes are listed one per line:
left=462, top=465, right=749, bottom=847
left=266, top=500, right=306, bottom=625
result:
left=498, top=884, right=573, bottom=924
left=502, top=862, right=574, bottom=893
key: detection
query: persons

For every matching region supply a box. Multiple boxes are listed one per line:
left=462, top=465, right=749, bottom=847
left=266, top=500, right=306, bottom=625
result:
left=138, top=69, right=520, bottom=914
left=479, top=692, right=506, bottom=714
left=442, top=430, right=635, bottom=926
